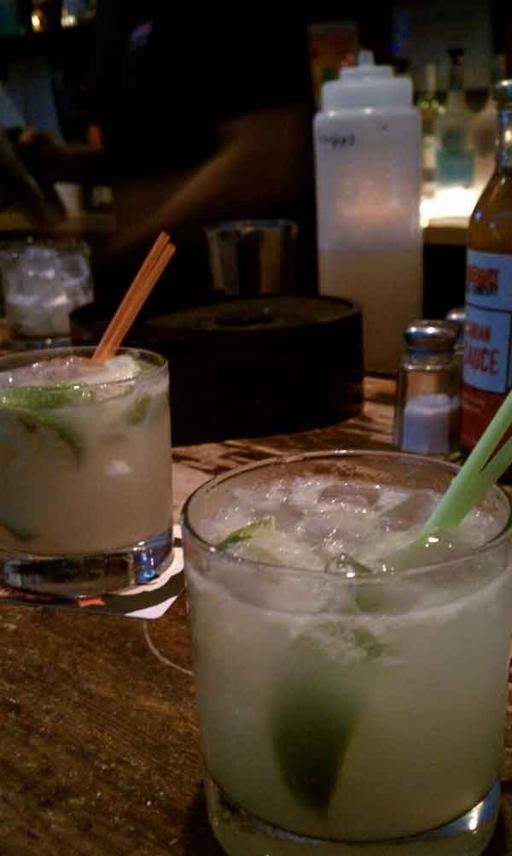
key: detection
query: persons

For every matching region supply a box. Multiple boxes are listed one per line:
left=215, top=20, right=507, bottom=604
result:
left=15, top=2, right=316, bottom=352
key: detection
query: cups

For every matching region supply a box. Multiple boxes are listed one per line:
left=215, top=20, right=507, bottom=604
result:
left=0, top=346, right=185, bottom=602
left=181, top=445, right=512, bottom=856
left=7, top=234, right=94, bottom=339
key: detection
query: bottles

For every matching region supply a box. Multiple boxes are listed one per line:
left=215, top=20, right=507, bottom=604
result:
left=314, top=52, right=512, bottom=460
